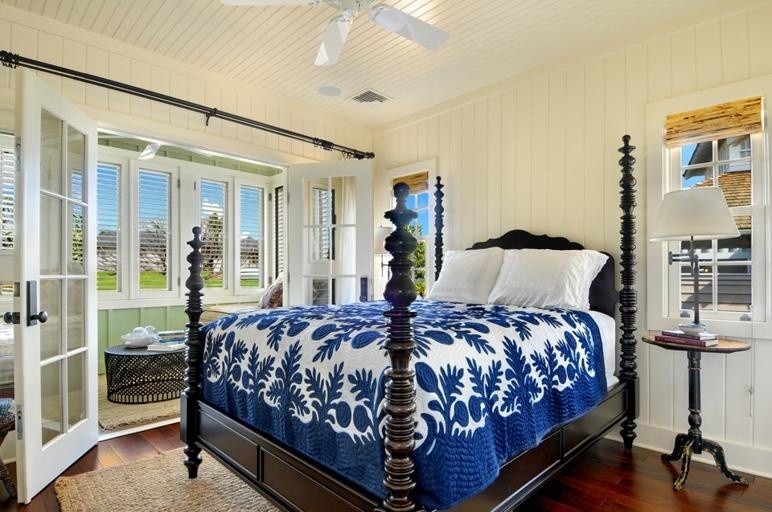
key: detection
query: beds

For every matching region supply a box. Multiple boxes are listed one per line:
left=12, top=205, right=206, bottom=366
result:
left=178, top=228, right=639, bottom=511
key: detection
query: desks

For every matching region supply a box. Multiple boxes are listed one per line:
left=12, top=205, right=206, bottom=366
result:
left=642, top=334, right=758, bottom=490
left=104, top=344, right=186, bottom=405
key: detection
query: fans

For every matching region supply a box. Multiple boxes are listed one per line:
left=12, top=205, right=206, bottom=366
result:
left=223, top=0, right=450, bottom=69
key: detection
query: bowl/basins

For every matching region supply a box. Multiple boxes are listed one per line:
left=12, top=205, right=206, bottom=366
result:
left=677, top=323, right=705, bottom=336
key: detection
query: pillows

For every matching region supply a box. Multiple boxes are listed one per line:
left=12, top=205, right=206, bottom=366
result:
left=422, top=247, right=610, bottom=311
left=257, top=266, right=329, bottom=306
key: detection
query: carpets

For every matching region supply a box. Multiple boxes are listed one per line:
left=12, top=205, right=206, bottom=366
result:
left=91, top=374, right=180, bottom=431
left=53, top=444, right=281, bottom=511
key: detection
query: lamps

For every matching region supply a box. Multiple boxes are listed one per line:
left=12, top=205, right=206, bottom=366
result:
left=647, top=186, right=741, bottom=323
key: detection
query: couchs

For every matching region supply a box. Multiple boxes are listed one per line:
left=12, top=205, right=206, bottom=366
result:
left=196, top=258, right=338, bottom=326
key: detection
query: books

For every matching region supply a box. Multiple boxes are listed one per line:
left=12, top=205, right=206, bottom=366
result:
left=653, top=329, right=720, bottom=347
left=146, top=328, right=189, bottom=352
